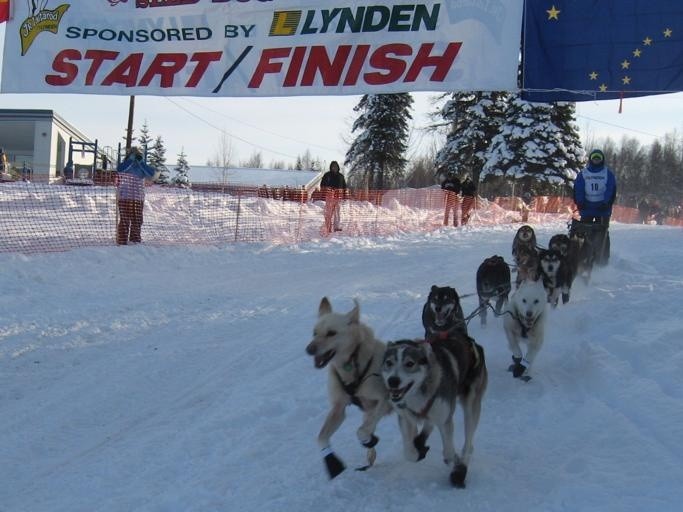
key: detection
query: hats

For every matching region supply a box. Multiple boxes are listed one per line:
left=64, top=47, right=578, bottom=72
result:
left=591, top=153, right=603, bottom=161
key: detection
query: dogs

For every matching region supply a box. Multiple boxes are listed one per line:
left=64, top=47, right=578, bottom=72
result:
left=475, top=224, right=597, bottom=384
left=378, top=333, right=489, bottom=490
left=302, top=294, right=400, bottom=482
left=419, top=282, right=470, bottom=339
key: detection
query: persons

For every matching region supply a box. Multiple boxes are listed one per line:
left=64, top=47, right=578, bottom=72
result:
left=319, top=161, right=348, bottom=233
left=297, top=161, right=303, bottom=170
left=310, top=162, right=315, bottom=170
left=573, top=149, right=616, bottom=266
left=461, top=177, right=477, bottom=225
left=440, top=170, right=460, bottom=227
left=520, top=183, right=683, bottom=226
left=115, top=146, right=156, bottom=244
